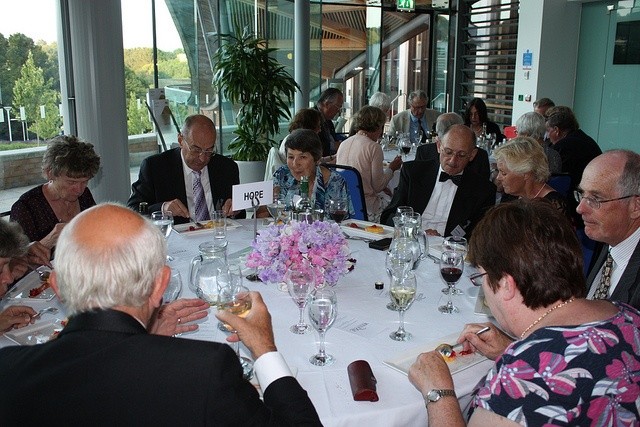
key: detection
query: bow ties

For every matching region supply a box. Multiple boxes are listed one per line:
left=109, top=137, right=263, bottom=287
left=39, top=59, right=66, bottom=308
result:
left=438, top=170, right=463, bottom=186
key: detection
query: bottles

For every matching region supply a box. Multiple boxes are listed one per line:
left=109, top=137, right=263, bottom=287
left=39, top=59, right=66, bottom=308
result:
left=297, top=176, right=312, bottom=213
left=480, top=122, right=488, bottom=141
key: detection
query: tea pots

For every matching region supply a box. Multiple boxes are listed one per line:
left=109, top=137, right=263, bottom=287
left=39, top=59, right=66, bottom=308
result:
left=386, top=217, right=428, bottom=270
left=189, top=239, right=229, bottom=305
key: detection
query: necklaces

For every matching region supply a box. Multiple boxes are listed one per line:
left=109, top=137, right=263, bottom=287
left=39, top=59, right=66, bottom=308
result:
left=520, top=297, right=575, bottom=338
left=534, top=182, right=546, bottom=198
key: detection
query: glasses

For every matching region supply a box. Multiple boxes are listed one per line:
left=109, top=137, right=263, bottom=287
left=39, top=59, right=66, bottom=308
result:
left=468, top=271, right=487, bottom=287
left=440, top=143, right=471, bottom=159
left=183, top=137, right=218, bottom=157
left=574, top=191, right=635, bottom=209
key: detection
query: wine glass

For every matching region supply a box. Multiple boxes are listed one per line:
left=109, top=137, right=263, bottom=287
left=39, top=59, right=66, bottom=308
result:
left=216, top=288, right=253, bottom=375
left=385, top=249, right=414, bottom=311
left=307, top=289, right=336, bottom=366
left=163, top=268, right=182, bottom=338
left=266, top=204, right=285, bottom=224
left=287, top=268, right=316, bottom=334
left=151, top=211, right=175, bottom=263
left=380, top=129, right=434, bottom=160
left=216, top=265, right=243, bottom=330
left=389, top=270, right=416, bottom=342
left=442, top=237, right=467, bottom=295
left=438, top=251, right=464, bottom=314
left=329, top=199, right=348, bottom=239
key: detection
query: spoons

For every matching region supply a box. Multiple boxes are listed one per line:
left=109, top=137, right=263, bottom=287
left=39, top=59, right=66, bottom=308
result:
left=27, top=263, right=50, bottom=281
left=436, top=327, right=490, bottom=358
left=189, top=216, right=204, bottom=228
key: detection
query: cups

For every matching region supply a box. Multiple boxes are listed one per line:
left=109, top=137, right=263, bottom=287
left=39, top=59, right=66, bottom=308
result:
left=211, top=210, right=227, bottom=240
left=402, top=212, right=421, bottom=225
left=397, top=206, right=413, bottom=223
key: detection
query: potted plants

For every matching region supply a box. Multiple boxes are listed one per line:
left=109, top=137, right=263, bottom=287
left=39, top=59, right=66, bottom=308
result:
left=204, top=24, right=303, bottom=185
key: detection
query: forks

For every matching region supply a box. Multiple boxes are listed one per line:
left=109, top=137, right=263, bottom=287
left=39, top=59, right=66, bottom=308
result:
left=31, top=307, right=58, bottom=318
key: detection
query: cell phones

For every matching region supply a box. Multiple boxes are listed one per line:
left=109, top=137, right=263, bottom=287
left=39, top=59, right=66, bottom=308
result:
left=368, top=236, right=393, bottom=252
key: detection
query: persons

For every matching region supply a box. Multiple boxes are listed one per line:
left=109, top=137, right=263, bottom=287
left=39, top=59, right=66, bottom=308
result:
left=545, top=105, right=602, bottom=175
left=0, top=201, right=324, bottom=427
left=311, top=87, right=341, bottom=156
left=491, top=134, right=576, bottom=231
left=10, top=134, right=100, bottom=261
left=278, top=108, right=337, bottom=166
left=464, top=98, right=503, bottom=143
left=390, top=89, right=442, bottom=142
left=408, top=196, right=640, bottom=427
left=533, top=98, right=555, bottom=115
left=127, top=114, right=247, bottom=225
left=13, top=240, right=52, bottom=281
left=336, top=106, right=402, bottom=223
left=369, top=92, right=391, bottom=142
left=380, top=123, right=496, bottom=247
left=415, top=113, right=491, bottom=182
left=256, top=129, right=351, bottom=221
left=573, top=148, right=640, bottom=312
left=0, top=217, right=41, bottom=334
left=516, top=111, right=562, bottom=174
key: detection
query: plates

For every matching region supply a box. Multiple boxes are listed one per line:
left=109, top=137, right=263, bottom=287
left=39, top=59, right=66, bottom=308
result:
left=474, top=286, right=495, bottom=317
left=381, top=330, right=488, bottom=378
left=428, top=235, right=465, bottom=260
left=4, top=318, right=65, bottom=346
left=340, top=219, right=395, bottom=241
left=4, top=266, right=56, bottom=302
left=172, top=218, right=242, bottom=234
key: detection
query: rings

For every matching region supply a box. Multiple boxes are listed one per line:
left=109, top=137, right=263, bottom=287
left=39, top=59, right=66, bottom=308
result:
left=177, top=317, right=182, bottom=325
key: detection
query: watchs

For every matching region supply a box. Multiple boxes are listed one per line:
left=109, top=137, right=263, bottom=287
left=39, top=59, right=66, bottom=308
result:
left=424, top=389, right=457, bottom=408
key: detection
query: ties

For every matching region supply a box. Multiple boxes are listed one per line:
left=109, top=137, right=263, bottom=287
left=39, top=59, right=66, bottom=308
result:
left=418, top=118, right=427, bottom=143
left=591, top=248, right=614, bottom=300
left=192, top=170, right=210, bottom=222
